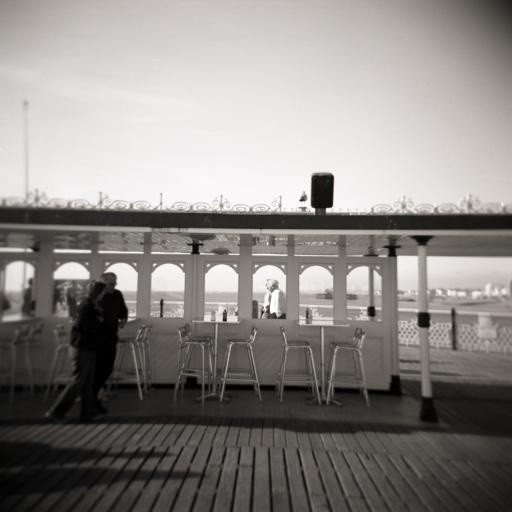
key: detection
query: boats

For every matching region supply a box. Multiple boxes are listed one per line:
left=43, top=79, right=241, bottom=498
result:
left=316, top=289, right=357, bottom=300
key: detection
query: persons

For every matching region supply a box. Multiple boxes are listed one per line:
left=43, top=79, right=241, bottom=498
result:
left=262, top=278, right=273, bottom=319
left=89, top=271, right=130, bottom=415
left=40, top=279, right=114, bottom=420
left=267, top=279, right=286, bottom=319
left=17, top=276, right=92, bottom=321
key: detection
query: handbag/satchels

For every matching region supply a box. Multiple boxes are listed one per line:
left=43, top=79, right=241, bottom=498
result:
left=70, top=325, right=104, bottom=352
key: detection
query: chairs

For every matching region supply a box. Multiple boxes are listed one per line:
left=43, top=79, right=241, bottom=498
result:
left=219, top=325, right=264, bottom=405
left=327, top=330, right=370, bottom=405
left=43, top=324, right=78, bottom=396
left=117, top=325, right=151, bottom=400
left=172, top=325, right=213, bottom=405
left=276, top=322, right=322, bottom=408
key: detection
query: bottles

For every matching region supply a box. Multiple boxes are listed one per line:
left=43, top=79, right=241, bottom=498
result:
left=211, top=309, right=216, bottom=321
left=223, top=309, right=227, bottom=321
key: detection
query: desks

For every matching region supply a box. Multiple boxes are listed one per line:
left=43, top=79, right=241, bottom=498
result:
left=294, top=317, right=350, bottom=408
left=192, top=317, right=241, bottom=406
